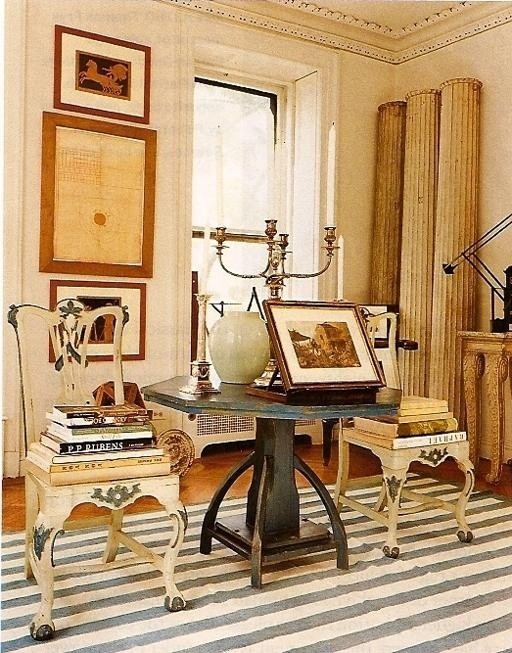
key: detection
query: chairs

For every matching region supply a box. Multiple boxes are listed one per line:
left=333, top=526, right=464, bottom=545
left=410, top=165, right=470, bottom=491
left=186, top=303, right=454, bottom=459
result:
left=7, top=300, right=188, bottom=642
left=328, top=306, right=475, bottom=558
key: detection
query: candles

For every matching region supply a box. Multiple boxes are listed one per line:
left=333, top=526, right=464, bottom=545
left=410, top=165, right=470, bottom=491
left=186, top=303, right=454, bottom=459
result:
left=215, top=121, right=336, bottom=227
left=201, top=220, right=210, bottom=296
left=337, top=234, right=344, bottom=299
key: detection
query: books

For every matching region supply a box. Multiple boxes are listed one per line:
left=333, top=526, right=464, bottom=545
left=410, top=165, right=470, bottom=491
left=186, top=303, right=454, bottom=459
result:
left=340, top=392, right=468, bottom=451
left=23, top=402, right=172, bottom=487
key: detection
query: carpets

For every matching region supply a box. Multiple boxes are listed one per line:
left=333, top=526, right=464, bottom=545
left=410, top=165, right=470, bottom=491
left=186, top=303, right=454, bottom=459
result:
left=2, top=472, right=512, bottom=653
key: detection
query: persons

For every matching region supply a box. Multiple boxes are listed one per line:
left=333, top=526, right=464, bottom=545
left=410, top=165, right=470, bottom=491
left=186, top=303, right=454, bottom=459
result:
left=309, top=337, right=319, bottom=354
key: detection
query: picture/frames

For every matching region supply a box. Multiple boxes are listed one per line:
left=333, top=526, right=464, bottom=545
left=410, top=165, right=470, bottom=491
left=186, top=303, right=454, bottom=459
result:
left=244, top=300, right=387, bottom=403
left=54, top=25, right=152, bottom=124
left=50, top=280, right=146, bottom=363
left=39, top=111, right=157, bottom=278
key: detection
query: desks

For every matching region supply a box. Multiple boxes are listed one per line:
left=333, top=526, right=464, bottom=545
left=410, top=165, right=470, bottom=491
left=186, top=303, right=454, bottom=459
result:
left=458, top=330, right=512, bottom=486
left=142, top=374, right=400, bottom=589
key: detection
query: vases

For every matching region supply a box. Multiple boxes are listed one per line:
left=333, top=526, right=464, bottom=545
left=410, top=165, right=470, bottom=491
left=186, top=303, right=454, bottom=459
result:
left=207, top=311, right=272, bottom=385
